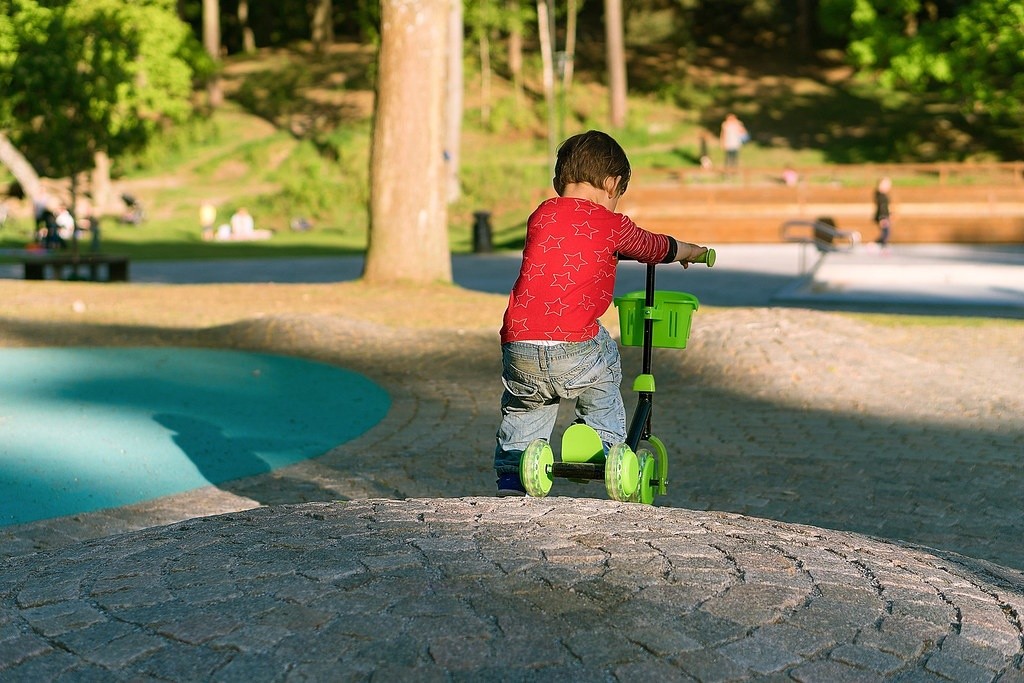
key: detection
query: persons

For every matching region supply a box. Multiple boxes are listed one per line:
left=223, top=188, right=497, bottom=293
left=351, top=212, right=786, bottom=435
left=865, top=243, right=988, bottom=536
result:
left=1, top=179, right=253, bottom=244
left=874, top=178, right=892, bottom=245
left=721, top=112, right=746, bottom=168
left=493, top=129, right=708, bottom=497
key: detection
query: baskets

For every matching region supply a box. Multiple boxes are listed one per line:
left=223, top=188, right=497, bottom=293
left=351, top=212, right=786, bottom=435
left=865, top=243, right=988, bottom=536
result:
left=614, top=290, right=699, bottom=349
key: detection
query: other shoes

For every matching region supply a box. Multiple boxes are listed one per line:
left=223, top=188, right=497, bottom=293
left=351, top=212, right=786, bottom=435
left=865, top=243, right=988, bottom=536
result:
left=495, top=472, right=527, bottom=495
left=571, top=418, right=611, bottom=458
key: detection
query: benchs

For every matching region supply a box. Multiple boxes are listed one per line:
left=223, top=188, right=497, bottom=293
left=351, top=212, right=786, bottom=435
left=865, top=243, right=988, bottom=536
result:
left=21, top=251, right=132, bottom=281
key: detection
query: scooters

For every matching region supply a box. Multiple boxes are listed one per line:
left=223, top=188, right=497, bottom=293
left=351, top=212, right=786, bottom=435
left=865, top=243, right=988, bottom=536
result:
left=520, top=246, right=717, bottom=512
